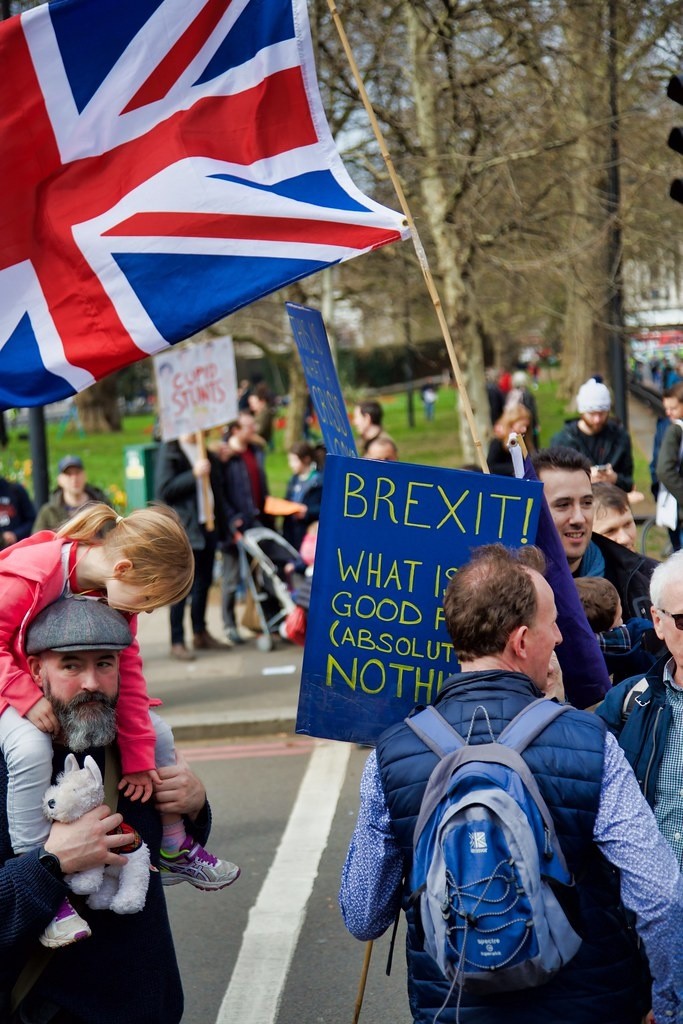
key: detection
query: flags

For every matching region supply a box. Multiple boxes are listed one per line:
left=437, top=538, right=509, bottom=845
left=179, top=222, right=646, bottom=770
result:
left=1, top=0, right=413, bottom=411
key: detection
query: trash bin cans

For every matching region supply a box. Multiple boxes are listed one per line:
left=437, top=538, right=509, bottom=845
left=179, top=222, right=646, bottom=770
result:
left=123, top=443, right=159, bottom=513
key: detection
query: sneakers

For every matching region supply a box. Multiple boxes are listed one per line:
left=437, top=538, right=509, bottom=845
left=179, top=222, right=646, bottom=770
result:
left=39, top=893, right=92, bottom=948
left=159, top=836, right=241, bottom=891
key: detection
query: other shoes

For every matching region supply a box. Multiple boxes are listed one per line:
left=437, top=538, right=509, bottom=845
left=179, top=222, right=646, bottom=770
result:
left=225, top=628, right=244, bottom=644
left=193, top=631, right=231, bottom=649
left=170, top=644, right=197, bottom=660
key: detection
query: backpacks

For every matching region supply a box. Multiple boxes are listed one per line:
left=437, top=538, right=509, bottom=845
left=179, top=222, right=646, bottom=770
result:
left=403, top=698, right=591, bottom=991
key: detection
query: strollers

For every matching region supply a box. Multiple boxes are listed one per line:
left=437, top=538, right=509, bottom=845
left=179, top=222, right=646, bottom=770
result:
left=227, top=507, right=311, bottom=652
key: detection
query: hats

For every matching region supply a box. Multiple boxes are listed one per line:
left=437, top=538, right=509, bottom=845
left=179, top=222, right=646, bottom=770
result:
left=58, top=454, right=83, bottom=474
left=25, top=596, right=133, bottom=652
left=575, top=375, right=611, bottom=414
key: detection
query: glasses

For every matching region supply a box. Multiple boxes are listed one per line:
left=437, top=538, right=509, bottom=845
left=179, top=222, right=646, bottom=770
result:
left=657, top=607, right=683, bottom=631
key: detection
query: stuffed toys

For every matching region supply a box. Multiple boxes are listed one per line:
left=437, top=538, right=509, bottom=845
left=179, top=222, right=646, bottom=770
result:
left=42, top=754, right=161, bottom=914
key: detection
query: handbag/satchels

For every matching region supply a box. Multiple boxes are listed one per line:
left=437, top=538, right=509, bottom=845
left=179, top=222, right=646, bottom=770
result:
left=656, top=481, right=678, bottom=530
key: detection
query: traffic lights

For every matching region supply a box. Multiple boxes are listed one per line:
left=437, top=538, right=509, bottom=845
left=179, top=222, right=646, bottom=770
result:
left=666, top=70, right=683, bottom=207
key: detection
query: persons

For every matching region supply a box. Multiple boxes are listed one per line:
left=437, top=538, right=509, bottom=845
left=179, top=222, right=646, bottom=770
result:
left=530, top=447, right=672, bottom=678
left=340, top=542, right=683, bottom=1024
left=594, top=553, right=683, bottom=873
left=0, top=504, right=242, bottom=949
left=1, top=376, right=399, bottom=661
left=590, top=478, right=637, bottom=554
left=417, top=349, right=683, bottom=536
left=572, top=576, right=624, bottom=636
left=1, top=600, right=213, bottom=1024
